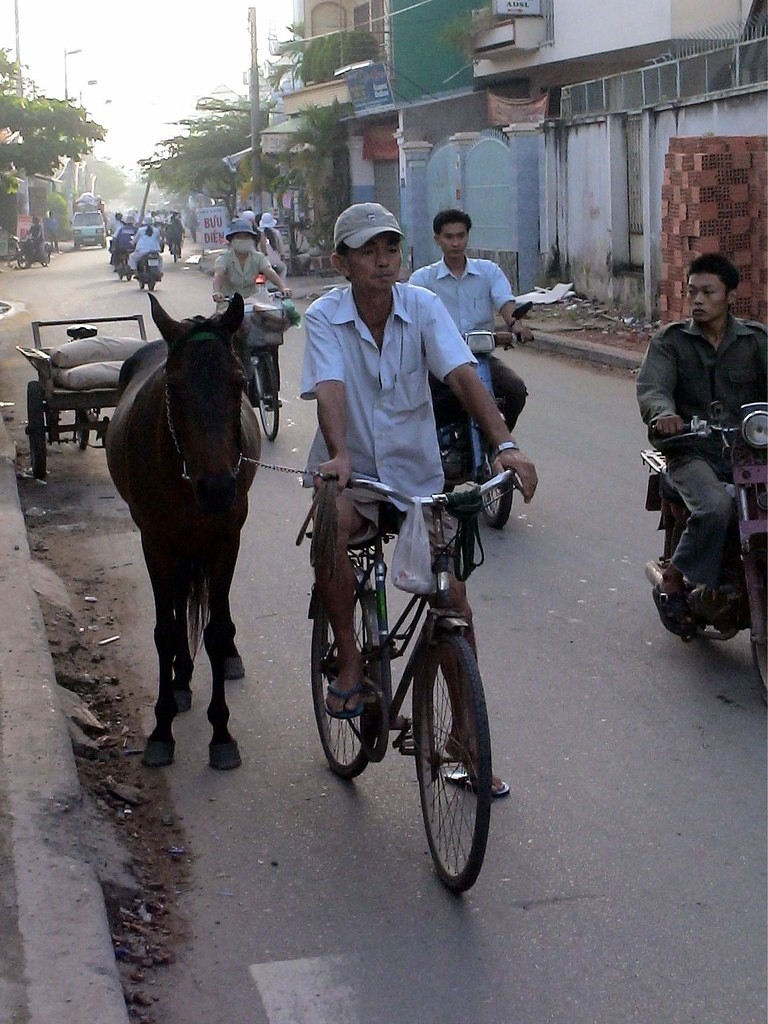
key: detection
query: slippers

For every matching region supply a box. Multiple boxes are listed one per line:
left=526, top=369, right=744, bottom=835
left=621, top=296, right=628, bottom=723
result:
left=324, top=682, right=363, bottom=718
left=445, top=772, right=510, bottom=796
left=655, top=583, right=691, bottom=626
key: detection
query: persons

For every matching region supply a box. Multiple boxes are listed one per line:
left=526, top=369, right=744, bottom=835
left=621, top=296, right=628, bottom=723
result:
left=212, top=217, right=293, bottom=409
left=301, top=202, right=510, bottom=797
left=147, top=210, right=152, bottom=216
left=128, top=216, right=161, bottom=275
left=188, top=210, right=199, bottom=243
left=108, top=213, right=124, bottom=252
left=113, top=216, right=138, bottom=273
left=408, top=209, right=529, bottom=466
left=45, top=209, right=62, bottom=255
left=22, top=215, right=43, bottom=256
left=241, top=210, right=288, bottom=284
left=636, top=252, right=768, bottom=616
left=150, top=208, right=185, bottom=258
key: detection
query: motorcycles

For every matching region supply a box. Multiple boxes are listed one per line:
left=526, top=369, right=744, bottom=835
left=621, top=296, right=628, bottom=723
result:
left=430, top=300, right=536, bottom=530
left=639, top=399, right=768, bottom=704
left=8, top=234, right=52, bottom=270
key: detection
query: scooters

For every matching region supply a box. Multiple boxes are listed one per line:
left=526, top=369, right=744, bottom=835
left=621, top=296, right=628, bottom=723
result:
left=107, top=220, right=186, bottom=291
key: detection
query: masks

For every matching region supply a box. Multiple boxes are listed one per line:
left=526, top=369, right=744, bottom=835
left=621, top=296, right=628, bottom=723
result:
left=230, top=238, right=253, bottom=255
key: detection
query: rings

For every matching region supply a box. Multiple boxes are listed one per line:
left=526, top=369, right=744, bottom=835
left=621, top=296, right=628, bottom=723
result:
left=286, top=291, right=290, bottom=293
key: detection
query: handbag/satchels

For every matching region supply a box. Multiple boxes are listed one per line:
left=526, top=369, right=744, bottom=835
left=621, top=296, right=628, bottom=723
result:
left=392, top=497, right=432, bottom=593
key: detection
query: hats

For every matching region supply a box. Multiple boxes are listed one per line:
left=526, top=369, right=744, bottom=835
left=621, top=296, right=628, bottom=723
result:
left=225, top=218, right=257, bottom=241
left=258, top=213, right=278, bottom=228
left=334, top=202, right=406, bottom=254
left=241, top=211, right=255, bottom=222
left=142, top=216, right=154, bottom=224
left=124, top=216, right=134, bottom=224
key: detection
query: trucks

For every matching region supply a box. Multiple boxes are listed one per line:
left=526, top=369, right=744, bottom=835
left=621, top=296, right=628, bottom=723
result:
left=69, top=209, right=107, bottom=250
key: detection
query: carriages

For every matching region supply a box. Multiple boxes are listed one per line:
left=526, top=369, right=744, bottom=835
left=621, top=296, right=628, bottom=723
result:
left=13, top=293, right=262, bottom=773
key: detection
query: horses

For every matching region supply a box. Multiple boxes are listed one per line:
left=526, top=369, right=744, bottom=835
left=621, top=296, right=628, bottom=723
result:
left=105, top=291, right=262, bottom=771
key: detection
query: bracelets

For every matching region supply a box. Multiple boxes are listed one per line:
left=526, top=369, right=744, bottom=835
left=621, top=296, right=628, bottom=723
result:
left=493, top=441, right=519, bottom=458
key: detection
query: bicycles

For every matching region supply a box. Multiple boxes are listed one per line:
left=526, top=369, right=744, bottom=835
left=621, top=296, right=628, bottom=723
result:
left=295, top=469, right=523, bottom=895
left=213, top=290, right=292, bottom=442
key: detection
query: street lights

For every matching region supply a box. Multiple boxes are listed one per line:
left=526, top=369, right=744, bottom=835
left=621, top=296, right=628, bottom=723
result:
left=75, top=79, right=98, bottom=198
left=62, top=47, right=83, bottom=226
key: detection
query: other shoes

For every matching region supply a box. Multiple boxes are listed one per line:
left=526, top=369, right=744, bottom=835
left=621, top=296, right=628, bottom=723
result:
left=269, top=400, right=284, bottom=409
